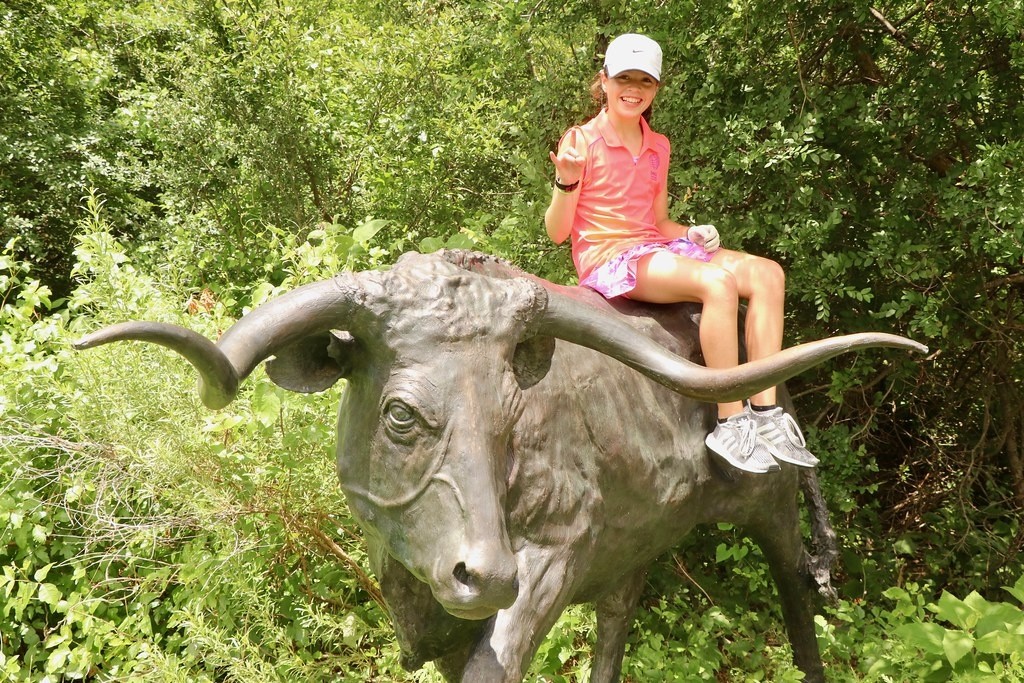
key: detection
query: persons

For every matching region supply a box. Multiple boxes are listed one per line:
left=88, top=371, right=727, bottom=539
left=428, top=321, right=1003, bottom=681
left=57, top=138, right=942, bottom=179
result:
left=544, top=32, right=822, bottom=475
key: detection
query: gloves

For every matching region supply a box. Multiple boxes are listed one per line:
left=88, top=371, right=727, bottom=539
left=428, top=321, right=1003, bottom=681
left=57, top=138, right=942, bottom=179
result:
left=686, top=223, right=720, bottom=253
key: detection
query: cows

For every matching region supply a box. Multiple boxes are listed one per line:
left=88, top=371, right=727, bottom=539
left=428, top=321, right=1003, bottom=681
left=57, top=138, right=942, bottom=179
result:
left=70, top=247, right=930, bottom=683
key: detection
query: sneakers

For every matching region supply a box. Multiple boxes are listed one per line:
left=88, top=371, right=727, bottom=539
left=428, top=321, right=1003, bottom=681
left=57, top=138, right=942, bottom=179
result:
left=705, top=413, right=782, bottom=473
left=744, top=399, right=820, bottom=468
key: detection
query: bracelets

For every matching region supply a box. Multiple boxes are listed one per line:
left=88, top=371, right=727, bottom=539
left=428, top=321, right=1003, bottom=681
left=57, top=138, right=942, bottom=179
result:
left=686, top=227, right=691, bottom=240
left=556, top=175, right=579, bottom=195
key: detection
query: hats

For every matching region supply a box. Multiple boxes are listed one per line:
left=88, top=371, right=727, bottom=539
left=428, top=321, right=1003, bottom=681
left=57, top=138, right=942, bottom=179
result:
left=603, top=34, right=662, bottom=82
